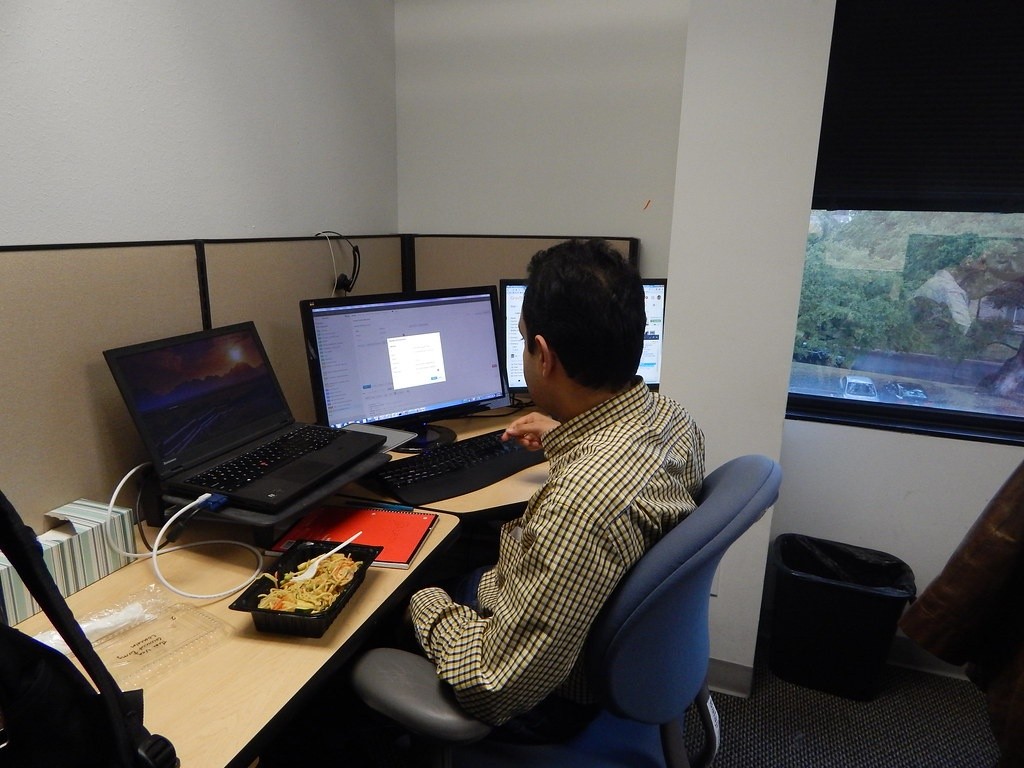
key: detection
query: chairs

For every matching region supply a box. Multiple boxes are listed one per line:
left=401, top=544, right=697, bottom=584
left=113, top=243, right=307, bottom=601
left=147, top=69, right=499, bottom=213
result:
left=307, top=455, right=782, bottom=767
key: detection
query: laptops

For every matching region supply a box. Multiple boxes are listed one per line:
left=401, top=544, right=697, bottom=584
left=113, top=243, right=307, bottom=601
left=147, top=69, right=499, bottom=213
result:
left=102, top=320, right=388, bottom=515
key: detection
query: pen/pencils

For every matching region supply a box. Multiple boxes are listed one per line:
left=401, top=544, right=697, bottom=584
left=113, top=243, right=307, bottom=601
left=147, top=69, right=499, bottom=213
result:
left=345, top=497, right=416, bottom=514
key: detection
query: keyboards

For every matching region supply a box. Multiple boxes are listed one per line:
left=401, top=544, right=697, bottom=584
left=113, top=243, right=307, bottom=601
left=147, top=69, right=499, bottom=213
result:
left=371, top=428, right=547, bottom=506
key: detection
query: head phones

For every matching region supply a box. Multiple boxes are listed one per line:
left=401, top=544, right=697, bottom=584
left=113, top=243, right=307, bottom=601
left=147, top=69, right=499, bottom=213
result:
left=315, top=231, right=361, bottom=292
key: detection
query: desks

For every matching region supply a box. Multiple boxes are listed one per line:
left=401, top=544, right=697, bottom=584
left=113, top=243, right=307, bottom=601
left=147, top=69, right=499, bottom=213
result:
left=0, top=494, right=460, bottom=768
left=333, top=400, right=663, bottom=512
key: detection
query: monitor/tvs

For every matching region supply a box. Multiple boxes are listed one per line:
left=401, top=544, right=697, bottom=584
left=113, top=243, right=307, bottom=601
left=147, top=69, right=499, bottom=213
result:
left=299, top=285, right=511, bottom=454
left=498, top=279, right=667, bottom=396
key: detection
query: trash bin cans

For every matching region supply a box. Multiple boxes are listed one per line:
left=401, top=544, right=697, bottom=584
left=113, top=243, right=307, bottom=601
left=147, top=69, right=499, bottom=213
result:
left=768, top=533, right=918, bottom=701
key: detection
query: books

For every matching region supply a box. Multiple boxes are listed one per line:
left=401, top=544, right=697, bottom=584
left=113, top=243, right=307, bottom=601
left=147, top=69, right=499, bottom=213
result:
left=256, top=492, right=414, bottom=555
left=264, top=504, right=440, bottom=570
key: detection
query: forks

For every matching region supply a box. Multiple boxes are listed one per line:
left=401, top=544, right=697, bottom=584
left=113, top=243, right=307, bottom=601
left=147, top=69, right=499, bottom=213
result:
left=288, top=531, right=364, bottom=581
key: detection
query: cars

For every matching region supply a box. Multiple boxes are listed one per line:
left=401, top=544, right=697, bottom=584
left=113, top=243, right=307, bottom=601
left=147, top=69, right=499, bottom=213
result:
left=881, top=380, right=929, bottom=406
left=839, top=375, right=879, bottom=402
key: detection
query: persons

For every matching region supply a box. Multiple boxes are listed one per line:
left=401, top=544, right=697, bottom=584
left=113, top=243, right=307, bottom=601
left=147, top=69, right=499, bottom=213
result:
left=345, top=237, right=706, bottom=745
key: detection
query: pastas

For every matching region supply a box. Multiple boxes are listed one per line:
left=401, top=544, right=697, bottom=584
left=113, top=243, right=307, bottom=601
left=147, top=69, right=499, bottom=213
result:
left=257, top=553, right=361, bottom=613
